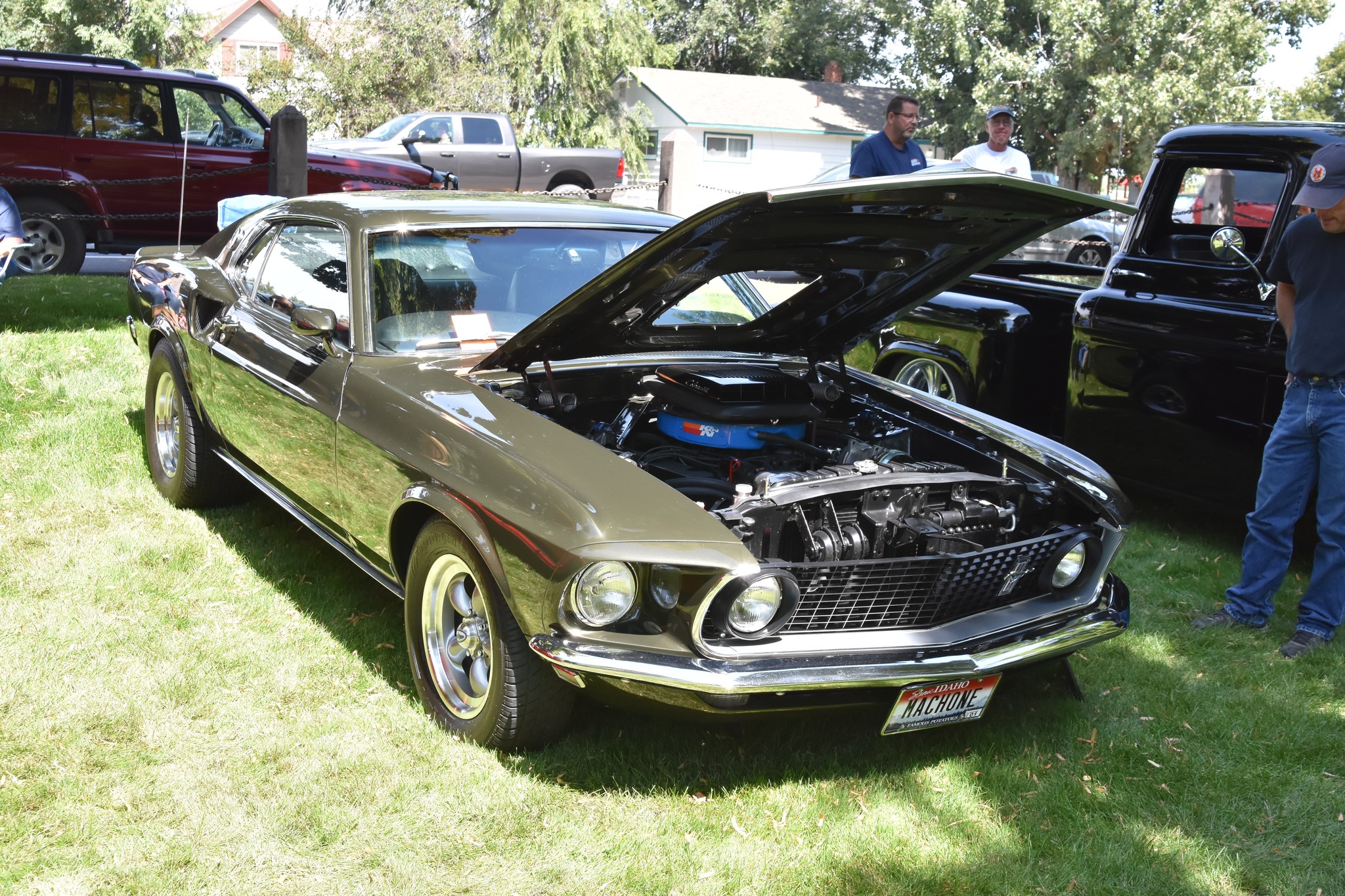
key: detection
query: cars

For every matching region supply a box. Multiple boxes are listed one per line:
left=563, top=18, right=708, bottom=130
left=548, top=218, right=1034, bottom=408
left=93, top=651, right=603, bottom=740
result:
left=1022, top=171, right=1124, bottom=267
left=1172, top=192, right=1200, bottom=224
left=119, top=165, right=1141, bottom=758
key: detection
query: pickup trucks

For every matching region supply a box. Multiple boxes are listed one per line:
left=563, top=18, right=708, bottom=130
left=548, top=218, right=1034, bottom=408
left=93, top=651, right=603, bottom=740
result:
left=312, top=111, right=626, bottom=203
left=859, top=116, right=1345, bottom=541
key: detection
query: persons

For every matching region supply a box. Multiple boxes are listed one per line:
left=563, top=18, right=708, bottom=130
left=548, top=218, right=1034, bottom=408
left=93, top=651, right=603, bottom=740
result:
left=952, top=105, right=1033, bottom=181
left=849, top=94, right=927, bottom=179
left=1193, top=141, right=1345, bottom=655
left=430, top=122, right=452, bottom=144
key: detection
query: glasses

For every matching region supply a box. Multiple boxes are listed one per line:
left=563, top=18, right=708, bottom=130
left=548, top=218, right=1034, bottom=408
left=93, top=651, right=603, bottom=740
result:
left=893, top=112, right=923, bottom=123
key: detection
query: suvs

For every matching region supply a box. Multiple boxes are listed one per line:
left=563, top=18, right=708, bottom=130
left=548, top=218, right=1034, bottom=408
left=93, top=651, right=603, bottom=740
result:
left=0, top=48, right=459, bottom=275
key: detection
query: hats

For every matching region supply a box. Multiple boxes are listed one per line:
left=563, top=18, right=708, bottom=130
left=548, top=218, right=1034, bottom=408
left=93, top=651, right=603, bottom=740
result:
left=986, top=105, right=1014, bottom=121
left=1291, top=143, right=1344, bottom=210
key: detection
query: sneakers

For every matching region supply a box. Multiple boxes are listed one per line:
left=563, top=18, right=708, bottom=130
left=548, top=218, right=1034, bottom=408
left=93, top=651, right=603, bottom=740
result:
left=1280, top=628, right=1329, bottom=660
left=1189, top=607, right=1268, bottom=632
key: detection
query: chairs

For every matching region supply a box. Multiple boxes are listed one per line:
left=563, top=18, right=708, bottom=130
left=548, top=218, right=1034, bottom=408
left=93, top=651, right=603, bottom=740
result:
left=503, top=261, right=598, bottom=315
left=121, top=103, right=163, bottom=141
left=1166, top=231, right=1217, bottom=262
left=374, top=257, right=436, bottom=323
